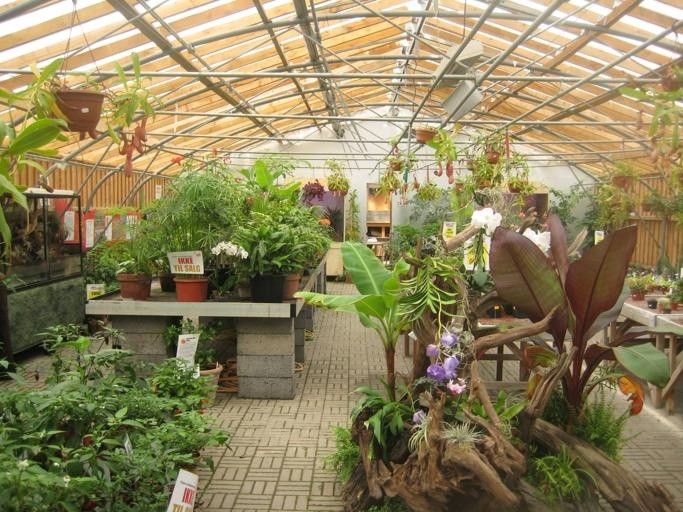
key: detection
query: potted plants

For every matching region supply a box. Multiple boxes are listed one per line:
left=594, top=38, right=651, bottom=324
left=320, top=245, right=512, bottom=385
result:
left=625, top=272, right=683, bottom=314
left=374, top=119, right=537, bottom=207
left=82, top=153, right=334, bottom=302
left=0, top=48, right=163, bottom=146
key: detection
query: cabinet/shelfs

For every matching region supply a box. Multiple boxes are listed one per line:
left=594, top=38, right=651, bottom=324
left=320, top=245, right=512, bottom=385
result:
left=3, top=194, right=84, bottom=295
